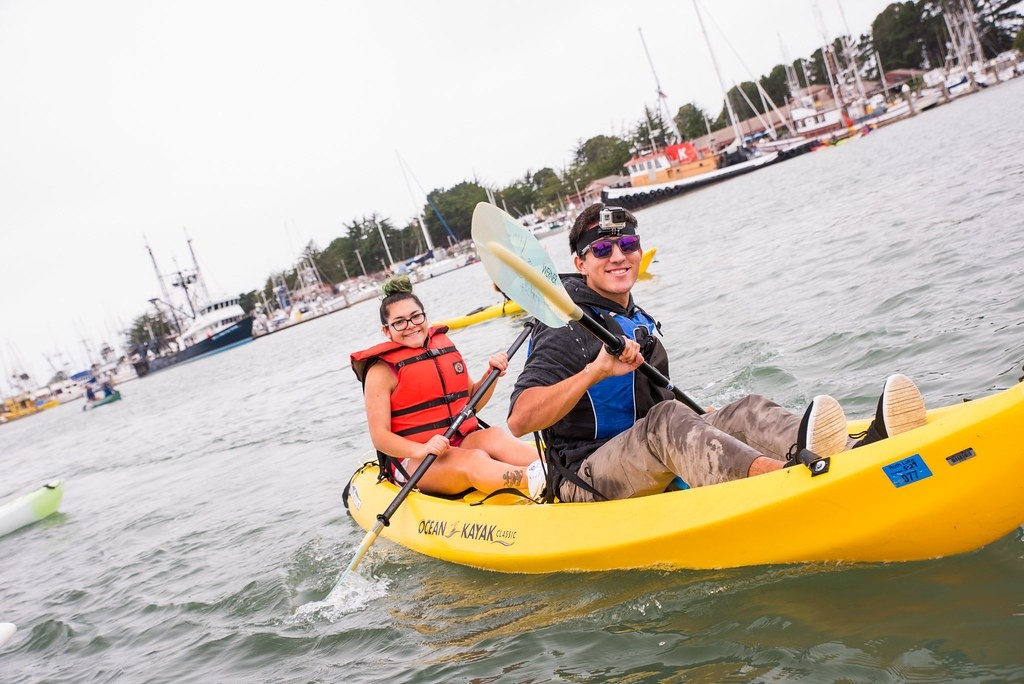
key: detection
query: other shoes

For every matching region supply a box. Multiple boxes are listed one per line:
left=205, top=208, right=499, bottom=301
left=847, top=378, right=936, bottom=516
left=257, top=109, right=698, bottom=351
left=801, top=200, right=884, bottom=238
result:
left=527, top=459, right=549, bottom=501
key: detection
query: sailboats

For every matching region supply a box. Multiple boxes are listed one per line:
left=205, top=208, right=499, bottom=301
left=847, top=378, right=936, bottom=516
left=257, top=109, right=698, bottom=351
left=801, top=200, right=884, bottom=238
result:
left=0, top=335, right=133, bottom=427
left=598, top=1, right=1023, bottom=205
left=248, top=133, right=578, bottom=339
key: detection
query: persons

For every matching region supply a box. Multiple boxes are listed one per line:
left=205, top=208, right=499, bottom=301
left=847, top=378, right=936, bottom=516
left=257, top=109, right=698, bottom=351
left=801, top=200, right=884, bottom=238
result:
left=507, top=203, right=926, bottom=503
left=351, top=280, right=550, bottom=504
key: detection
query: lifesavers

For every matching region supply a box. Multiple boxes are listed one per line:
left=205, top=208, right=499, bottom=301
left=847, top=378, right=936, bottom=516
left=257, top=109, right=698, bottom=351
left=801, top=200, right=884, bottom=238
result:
left=618, top=185, right=681, bottom=202
left=777, top=140, right=820, bottom=159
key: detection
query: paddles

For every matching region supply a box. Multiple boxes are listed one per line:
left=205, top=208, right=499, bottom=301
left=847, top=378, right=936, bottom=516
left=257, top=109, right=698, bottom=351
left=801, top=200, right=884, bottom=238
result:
left=470, top=202, right=706, bottom=416
left=324, top=316, right=540, bottom=601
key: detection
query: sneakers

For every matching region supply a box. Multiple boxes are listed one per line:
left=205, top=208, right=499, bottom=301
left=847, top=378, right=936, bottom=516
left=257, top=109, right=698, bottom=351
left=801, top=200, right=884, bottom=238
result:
left=849, top=373, right=929, bottom=449
left=782, top=394, right=850, bottom=471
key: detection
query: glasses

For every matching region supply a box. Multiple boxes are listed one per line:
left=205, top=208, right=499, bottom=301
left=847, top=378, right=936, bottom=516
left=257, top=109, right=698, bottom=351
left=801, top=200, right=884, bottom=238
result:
left=385, top=311, right=426, bottom=332
left=588, top=235, right=641, bottom=259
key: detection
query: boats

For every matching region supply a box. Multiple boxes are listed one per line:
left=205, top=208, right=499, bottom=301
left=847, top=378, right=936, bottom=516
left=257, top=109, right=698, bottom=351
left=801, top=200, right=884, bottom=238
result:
left=122, top=218, right=256, bottom=378
left=339, top=377, right=1024, bottom=578
left=82, top=390, right=121, bottom=413
left=0, top=477, right=68, bottom=539
